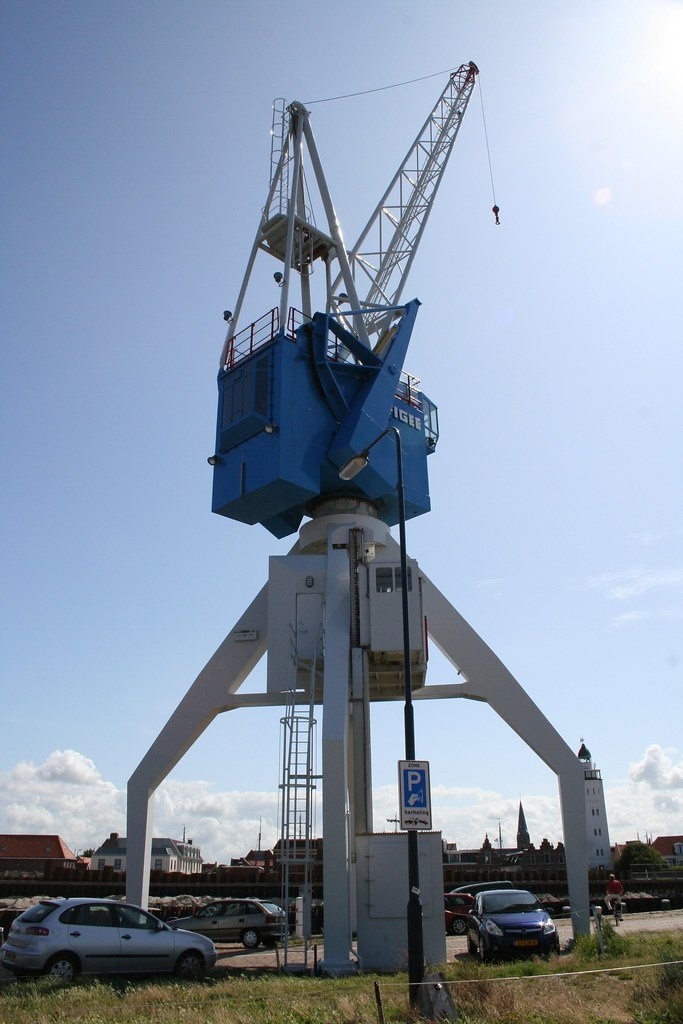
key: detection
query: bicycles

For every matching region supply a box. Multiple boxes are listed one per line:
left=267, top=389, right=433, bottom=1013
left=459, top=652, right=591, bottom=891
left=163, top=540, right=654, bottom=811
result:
left=610, top=898, right=624, bottom=927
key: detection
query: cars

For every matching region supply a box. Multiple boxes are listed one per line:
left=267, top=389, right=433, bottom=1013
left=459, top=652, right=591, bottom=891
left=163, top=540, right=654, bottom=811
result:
left=0, top=895, right=217, bottom=989
left=464, top=889, right=561, bottom=960
left=164, top=896, right=291, bottom=950
left=443, top=893, right=475, bottom=936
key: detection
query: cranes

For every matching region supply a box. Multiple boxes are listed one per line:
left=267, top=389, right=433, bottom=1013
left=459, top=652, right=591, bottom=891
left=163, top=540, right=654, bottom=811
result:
left=120, top=70, right=629, bottom=954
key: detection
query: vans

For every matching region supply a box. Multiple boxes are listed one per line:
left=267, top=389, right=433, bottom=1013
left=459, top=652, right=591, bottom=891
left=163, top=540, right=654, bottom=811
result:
left=449, top=880, right=514, bottom=897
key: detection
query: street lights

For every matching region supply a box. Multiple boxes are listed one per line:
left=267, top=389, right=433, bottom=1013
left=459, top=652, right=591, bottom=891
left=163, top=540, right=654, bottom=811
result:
left=494, top=817, right=504, bottom=881
left=385, top=813, right=400, bottom=833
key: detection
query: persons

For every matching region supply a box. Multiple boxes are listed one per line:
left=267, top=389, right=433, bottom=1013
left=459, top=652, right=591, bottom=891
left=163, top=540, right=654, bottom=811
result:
left=604, top=874, right=624, bottom=922
